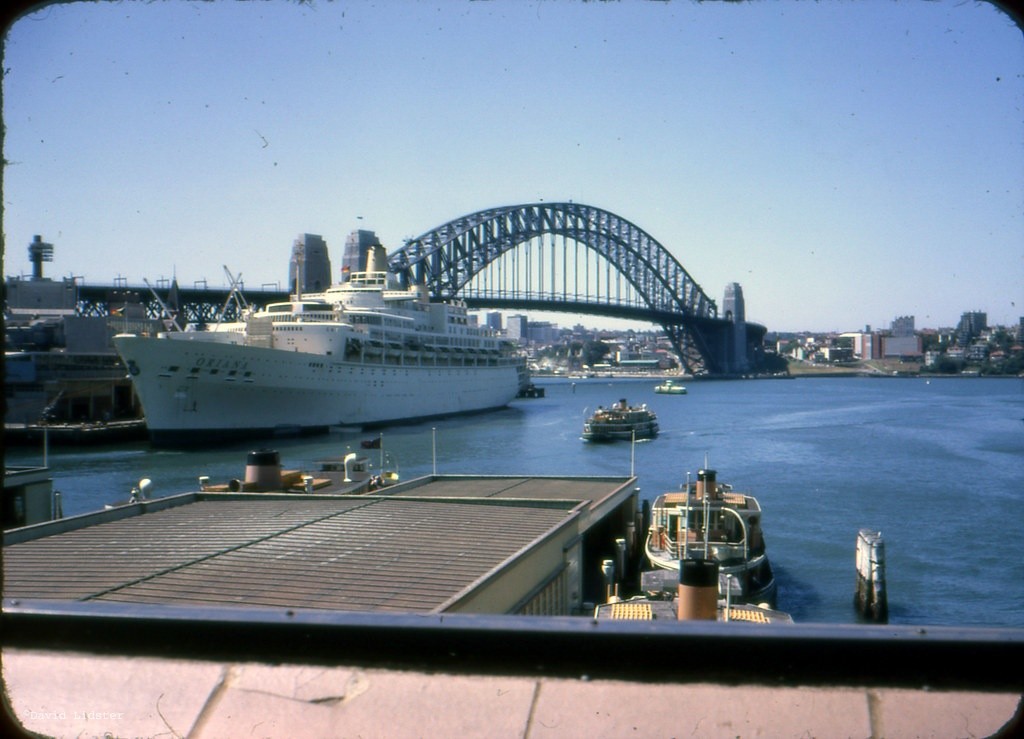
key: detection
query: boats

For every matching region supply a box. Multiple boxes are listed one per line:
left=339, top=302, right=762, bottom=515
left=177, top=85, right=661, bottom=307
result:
left=594, top=451, right=793, bottom=623
left=111, top=244, right=529, bottom=452
left=655, top=380, right=687, bottom=394
left=104, top=432, right=400, bottom=510
left=579, top=398, right=659, bottom=444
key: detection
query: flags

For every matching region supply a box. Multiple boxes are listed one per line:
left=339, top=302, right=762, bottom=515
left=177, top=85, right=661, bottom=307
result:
left=111, top=307, right=125, bottom=316
left=341, top=266, right=350, bottom=273
left=361, top=438, right=380, bottom=450
